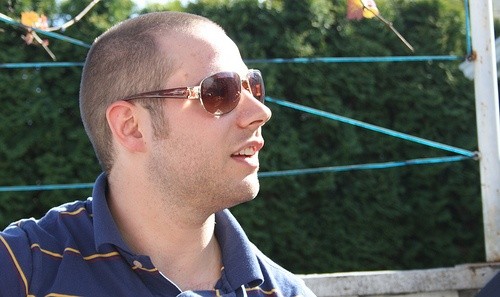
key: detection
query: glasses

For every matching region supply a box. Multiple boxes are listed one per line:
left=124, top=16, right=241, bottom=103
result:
left=123, top=68, right=265, bottom=116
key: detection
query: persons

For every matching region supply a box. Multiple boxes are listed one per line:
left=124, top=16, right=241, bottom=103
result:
left=0, top=10, right=317, bottom=297
